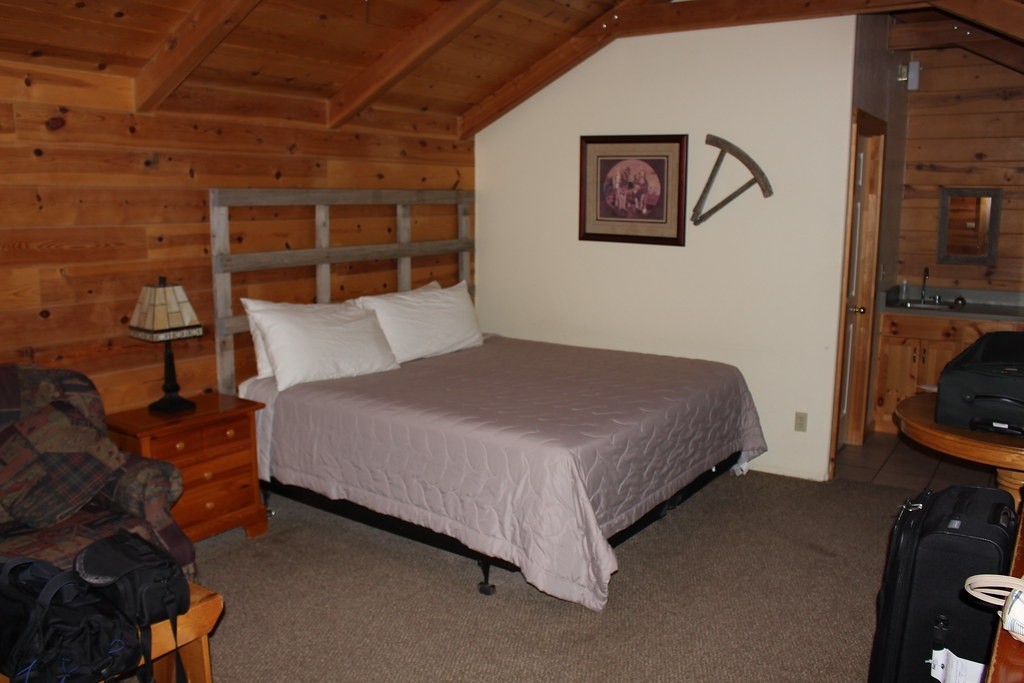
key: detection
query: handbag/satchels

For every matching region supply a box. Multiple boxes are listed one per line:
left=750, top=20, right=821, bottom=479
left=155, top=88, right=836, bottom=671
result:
left=74, top=528, right=191, bottom=626
left=964, top=575, right=1024, bottom=644
left=935, top=331, right=1024, bottom=437
left=0, top=556, right=142, bottom=683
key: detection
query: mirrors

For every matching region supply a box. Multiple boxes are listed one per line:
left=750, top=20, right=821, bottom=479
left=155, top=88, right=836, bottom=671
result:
left=938, top=187, right=1002, bottom=267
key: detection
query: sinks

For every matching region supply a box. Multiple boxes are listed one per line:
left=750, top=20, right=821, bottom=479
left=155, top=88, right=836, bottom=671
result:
left=900, top=303, right=955, bottom=311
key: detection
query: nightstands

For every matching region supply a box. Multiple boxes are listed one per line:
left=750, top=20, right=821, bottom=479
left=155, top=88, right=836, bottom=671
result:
left=105, top=393, right=270, bottom=542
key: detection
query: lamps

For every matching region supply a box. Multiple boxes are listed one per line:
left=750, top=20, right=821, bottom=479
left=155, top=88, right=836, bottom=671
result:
left=126, top=276, right=204, bottom=419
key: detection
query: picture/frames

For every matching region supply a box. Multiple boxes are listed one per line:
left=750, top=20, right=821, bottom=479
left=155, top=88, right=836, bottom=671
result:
left=578, top=134, right=688, bottom=247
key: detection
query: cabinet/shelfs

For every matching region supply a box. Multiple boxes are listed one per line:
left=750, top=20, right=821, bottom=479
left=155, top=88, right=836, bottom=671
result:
left=871, top=312, right=1024, bottom=434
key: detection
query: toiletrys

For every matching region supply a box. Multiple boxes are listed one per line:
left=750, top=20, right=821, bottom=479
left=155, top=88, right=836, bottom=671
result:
left=899, top=278, right=910, bottom=300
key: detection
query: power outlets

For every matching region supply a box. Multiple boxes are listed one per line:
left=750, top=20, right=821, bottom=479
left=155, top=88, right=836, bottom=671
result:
left=795, top=411, right=808, bottom=432
left=208, top=186, right=770, bottom=616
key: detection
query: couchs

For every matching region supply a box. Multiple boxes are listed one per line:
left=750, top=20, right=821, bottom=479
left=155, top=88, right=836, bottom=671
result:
left=0, top=362, right=196, bottom=583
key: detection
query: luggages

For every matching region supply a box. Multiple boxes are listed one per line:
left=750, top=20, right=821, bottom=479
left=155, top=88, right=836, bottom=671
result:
left=868, top=484, right=1018, bottom=683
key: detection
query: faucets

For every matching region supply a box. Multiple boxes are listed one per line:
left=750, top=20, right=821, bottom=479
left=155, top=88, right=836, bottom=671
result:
left=921, top=267, right=930, bottom=305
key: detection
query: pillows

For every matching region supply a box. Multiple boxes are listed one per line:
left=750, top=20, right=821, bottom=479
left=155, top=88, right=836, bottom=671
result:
left=250, top=308, right=402, bottom=392
left=240, top=298, right=354, bottom=379
left=359, top=280, right=484, bottom=363
left=0, top=400, right=131, bottom=532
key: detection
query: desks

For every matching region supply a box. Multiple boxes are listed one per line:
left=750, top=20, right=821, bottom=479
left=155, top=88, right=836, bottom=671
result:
left=982, top=516, right=1024, bottom=683
left=893, top=393, right=1024, bottom=514
left=0, top=578, right=224, bottom=683
